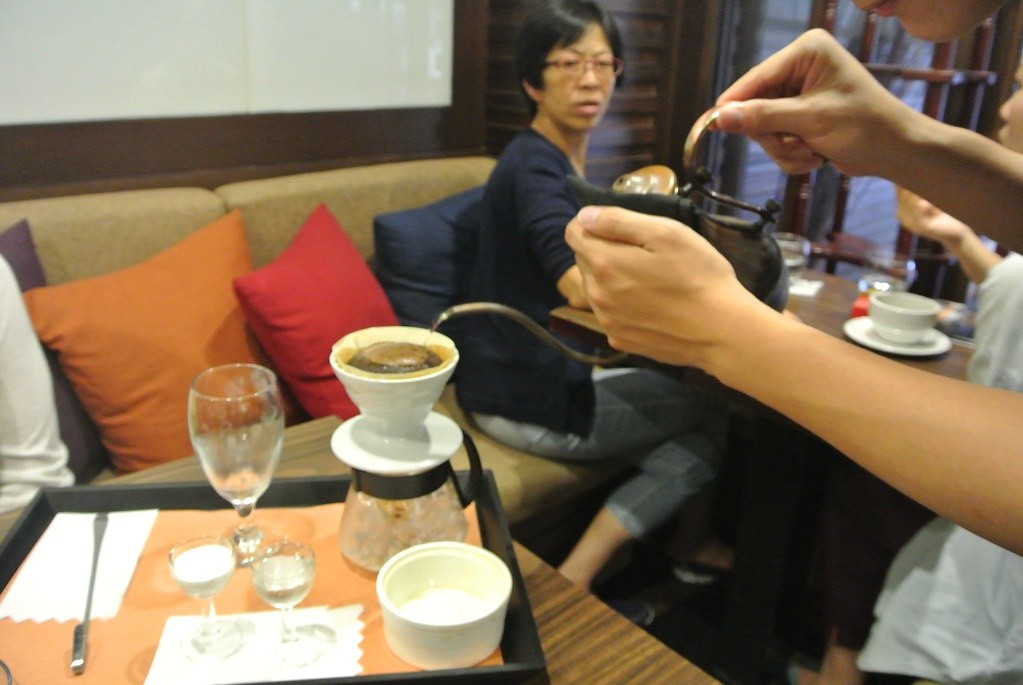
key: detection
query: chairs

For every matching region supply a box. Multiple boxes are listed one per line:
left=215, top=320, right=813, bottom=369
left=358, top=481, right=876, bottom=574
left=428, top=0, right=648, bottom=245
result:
left=0, top=221, right=109, bottom=508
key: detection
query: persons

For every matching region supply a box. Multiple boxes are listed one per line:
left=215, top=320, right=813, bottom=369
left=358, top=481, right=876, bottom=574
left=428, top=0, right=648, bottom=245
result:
left=851, top=52, right=1023, bottom=684
left=565, top=1, right=1023, bottom=558
left=449, top=0, right=735, bottom=608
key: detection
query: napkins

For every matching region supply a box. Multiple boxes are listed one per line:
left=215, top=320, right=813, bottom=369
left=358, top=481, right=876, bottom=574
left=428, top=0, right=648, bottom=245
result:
left=0, top=506, right=160, bottom=622
left=143, top=604, right=365, bottom=684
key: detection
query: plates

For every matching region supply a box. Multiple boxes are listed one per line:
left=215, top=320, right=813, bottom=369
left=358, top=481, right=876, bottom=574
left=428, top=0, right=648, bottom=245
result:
left=843, top=315, right=953, bottom=355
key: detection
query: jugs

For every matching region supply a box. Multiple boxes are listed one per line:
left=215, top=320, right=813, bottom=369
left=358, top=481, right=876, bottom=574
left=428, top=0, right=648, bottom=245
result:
left=448, top=101, right=789, bottom=370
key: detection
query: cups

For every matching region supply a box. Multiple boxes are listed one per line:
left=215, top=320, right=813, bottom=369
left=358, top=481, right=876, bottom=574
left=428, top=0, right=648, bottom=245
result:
left=771, top=232, right=811, bottom=286
left=870, top=291, right=941, bottom=345
left=862, top=249, right=916, bottom=291
left=0, top=661, right=12, bottom=685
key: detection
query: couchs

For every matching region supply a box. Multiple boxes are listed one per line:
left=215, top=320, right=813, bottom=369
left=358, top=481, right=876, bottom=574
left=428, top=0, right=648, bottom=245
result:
left=0, top=149, right=631, bottom=529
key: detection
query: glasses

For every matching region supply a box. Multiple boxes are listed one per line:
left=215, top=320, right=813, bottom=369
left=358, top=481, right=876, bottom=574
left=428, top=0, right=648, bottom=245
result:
left=525, top=54, right=626, bottom=81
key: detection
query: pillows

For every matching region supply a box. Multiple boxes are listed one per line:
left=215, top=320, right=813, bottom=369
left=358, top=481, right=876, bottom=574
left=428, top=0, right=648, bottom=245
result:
left=21, top=208, right=294, bottom=476
left=373, top=182, right=494, bottom=384
left=235, top=200, right=401, bottom=421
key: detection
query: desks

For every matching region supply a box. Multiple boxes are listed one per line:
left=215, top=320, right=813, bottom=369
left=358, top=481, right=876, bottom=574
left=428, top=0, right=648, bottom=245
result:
left=553, top=252, right=977, bottom=378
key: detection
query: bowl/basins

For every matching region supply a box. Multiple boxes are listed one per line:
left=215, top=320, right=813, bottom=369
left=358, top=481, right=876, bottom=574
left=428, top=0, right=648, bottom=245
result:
left=377, top=541, right=512, bottom=671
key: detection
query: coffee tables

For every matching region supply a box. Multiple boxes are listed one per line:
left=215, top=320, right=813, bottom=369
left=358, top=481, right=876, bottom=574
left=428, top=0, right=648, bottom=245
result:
left=510, top=536, right=728, bottom=684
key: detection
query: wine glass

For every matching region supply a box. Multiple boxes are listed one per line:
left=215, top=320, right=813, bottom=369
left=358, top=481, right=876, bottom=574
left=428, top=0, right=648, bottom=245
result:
left=187, top=362, right=285, bottom=566
left=251, top=546, right=324, bottom=667
left=169, top=536, right=244, bottom=657
